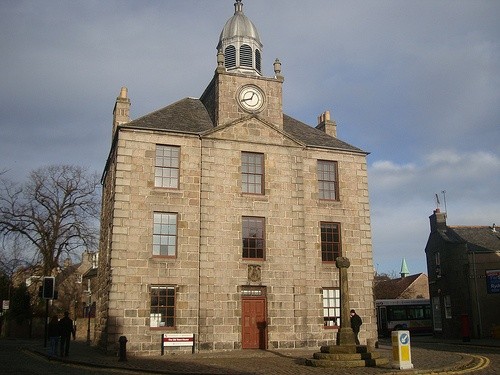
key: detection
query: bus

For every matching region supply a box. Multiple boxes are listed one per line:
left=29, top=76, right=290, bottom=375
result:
left=374, top=298, right=432, bottom=337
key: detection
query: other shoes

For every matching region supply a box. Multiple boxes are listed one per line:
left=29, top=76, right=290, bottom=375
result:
left=356, top=343, right=360, bottom=345
left=48, top=357, right=53, bottom=361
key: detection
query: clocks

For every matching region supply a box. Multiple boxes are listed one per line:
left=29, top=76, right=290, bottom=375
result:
left=238, top=86, right=264, bottom=111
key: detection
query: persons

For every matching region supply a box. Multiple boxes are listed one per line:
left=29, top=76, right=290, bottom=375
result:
left=60, top=312, right=76, bottom=360
left=48, top=315, right=61, bottom=361
left=350, top=309, right=362, bottom=345
left=461, top=316, right=471, bottom=345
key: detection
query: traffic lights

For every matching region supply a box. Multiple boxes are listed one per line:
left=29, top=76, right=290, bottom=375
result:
left=43, top=277, right=55, bottom=299
